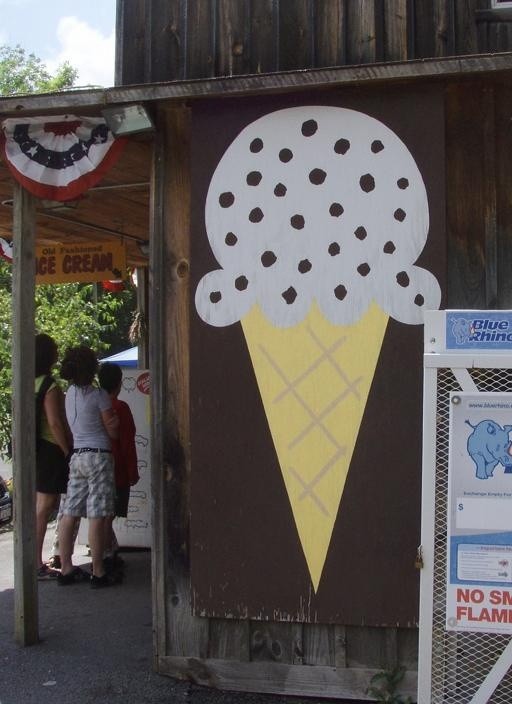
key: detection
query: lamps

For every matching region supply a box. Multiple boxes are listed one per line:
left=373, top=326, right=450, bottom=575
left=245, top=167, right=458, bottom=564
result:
left=101, top=101, right=156, bottom=140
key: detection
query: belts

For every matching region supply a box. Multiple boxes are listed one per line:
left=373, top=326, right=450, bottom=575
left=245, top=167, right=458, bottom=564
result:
left=70, top=448, right=111, bottom=455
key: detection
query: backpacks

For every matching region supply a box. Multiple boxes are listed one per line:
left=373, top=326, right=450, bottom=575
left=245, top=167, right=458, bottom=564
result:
left=6, top=376, right=55, bottom=460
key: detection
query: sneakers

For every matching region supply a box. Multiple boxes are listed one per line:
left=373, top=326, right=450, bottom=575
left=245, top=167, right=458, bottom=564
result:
left=37, top=555, right=123, bottom=589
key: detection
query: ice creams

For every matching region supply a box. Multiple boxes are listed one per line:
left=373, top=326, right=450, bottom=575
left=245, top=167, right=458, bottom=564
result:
left=195, top=103, right=442, bottom=595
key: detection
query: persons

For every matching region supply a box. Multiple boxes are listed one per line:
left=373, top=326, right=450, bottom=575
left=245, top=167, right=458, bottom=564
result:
left=34, top=332, right=72, bottom=581
left=54, top=345, right=123, bottom=589
left=88, top=359, right=142, bottom=580
left=44, top=492, right=117, bottom=572
